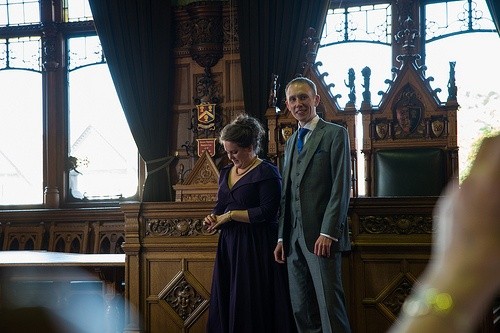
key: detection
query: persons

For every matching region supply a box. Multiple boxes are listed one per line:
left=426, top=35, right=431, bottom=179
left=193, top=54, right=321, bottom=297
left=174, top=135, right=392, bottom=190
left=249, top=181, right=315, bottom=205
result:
left=387, top=134, right=499, bottom=333
left=202, top=113, right=282, bottom=333
left=273, top=78, right=353, bottom=333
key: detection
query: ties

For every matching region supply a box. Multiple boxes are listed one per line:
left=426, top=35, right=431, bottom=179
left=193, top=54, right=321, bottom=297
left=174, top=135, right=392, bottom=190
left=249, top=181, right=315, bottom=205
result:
left=298, top=128, right=310, bottom=154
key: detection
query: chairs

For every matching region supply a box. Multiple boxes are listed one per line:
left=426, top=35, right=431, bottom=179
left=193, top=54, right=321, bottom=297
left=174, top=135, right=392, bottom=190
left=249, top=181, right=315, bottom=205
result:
left=2, top=221, right=125, bottom=309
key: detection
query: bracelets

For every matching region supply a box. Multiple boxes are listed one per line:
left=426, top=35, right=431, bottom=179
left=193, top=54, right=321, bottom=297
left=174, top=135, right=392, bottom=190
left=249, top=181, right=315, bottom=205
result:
left=412, top=280, right=470, bottom=330
left=228, top=210, right=232, bottom=221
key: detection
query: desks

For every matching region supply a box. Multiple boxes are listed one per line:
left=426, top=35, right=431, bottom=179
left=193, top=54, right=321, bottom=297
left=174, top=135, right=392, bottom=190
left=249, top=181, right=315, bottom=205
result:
left=0, top=251, right=126, bottom=333
